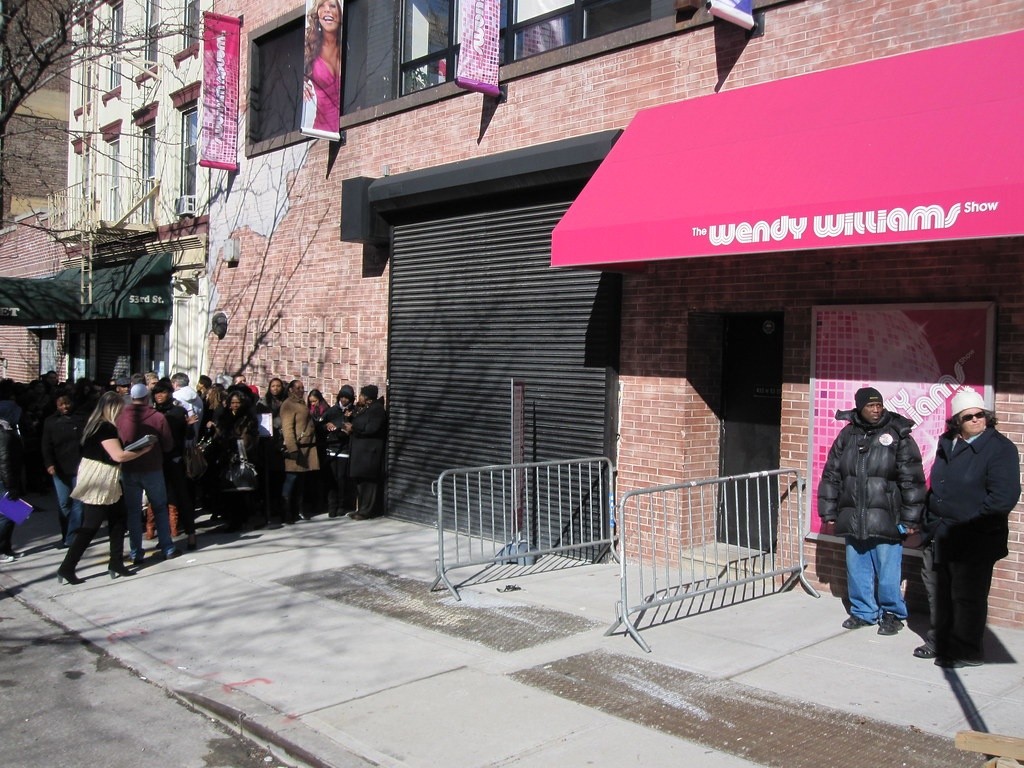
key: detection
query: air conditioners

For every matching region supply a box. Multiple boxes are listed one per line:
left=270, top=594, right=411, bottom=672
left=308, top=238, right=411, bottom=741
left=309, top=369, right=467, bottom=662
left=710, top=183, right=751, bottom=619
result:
left=175, top=196, right=194, bottom=217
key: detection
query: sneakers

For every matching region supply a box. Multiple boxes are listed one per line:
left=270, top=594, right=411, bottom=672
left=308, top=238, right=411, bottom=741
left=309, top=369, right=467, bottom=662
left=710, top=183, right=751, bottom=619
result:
left=877, top=610, right=904, bottom=635
left=842, top=615, right=868, bottom=629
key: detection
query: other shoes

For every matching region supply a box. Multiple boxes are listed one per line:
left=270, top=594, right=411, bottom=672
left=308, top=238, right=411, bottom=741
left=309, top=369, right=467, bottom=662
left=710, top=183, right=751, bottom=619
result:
left=0, top=553, right=14, bottom=562
left=349, top=513, right=366, bottom=520
left=56, top=543, right=70, bottom=550
left=11, top=552, right=25, bottom=558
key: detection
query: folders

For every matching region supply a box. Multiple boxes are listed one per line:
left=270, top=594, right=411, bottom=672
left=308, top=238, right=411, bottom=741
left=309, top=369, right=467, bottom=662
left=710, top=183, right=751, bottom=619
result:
left=0, top=491, right=33, bottom=526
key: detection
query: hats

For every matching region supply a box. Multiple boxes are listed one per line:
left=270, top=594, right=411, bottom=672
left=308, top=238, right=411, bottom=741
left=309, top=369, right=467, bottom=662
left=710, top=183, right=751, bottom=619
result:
left=116, top=377, right=131, bottom=386
left=130, top=383, right=149, bottom=399
left=950, top=386, right=988, bottom=418
left=855, top=388, right=883, bottom=413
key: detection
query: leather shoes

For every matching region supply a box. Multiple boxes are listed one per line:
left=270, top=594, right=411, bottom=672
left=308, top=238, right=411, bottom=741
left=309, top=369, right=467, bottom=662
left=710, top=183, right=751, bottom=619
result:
left=912, top=644, right=941, bottom=658
left=934, top=653, right=985, bottom=669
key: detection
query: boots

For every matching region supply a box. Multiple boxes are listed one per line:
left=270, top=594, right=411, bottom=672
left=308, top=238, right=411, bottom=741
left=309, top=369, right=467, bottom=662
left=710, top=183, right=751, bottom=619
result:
left=169, top=504, right=178, bottom=537
left=280, top=492, right=346, bottom=525
left=145, top=503, right=156, bottom=540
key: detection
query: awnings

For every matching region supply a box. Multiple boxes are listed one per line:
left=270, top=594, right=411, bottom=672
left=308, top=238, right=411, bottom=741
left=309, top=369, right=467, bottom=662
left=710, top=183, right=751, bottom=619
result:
left=0, top=253, right=172, bottom=325
left=548, top=27, right=1023, bottom=268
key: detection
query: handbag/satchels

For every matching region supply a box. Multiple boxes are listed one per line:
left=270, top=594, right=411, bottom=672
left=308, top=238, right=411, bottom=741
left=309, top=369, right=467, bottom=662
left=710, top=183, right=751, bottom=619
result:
left=348, top=438, right=384, bottom=480
left=185, top=442, right=208, bottom=478
left=324, top=428, right=348, bottom=445
left=228, top=439, right=257, bottom=489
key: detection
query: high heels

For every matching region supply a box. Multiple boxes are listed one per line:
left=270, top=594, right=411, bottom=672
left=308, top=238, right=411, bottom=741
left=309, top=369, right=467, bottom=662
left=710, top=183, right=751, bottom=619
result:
left=56, top=534, right=199, bottom=586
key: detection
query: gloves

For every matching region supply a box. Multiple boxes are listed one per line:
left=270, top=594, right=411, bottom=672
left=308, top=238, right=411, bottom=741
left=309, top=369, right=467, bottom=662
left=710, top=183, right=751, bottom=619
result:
left=312, top=406, right=320, bottom=418
left=290, top=451, right=298, bottom=460
left=7, top=487, right=19, bottom=500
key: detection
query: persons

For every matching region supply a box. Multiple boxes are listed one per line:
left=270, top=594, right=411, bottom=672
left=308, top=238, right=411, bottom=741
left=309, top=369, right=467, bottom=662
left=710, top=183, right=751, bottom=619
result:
left=0, top=370, right=389, bottom=583
left=817, top=386, right=929, bottom=636
left=302, top=1, right=342, bottom=134
left=913, top=390, right=1021, bottom=670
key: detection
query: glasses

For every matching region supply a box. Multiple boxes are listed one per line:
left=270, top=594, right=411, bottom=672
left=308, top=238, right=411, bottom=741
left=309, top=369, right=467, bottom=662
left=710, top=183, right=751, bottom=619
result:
left=959, top=411, right=986, bottom=424
